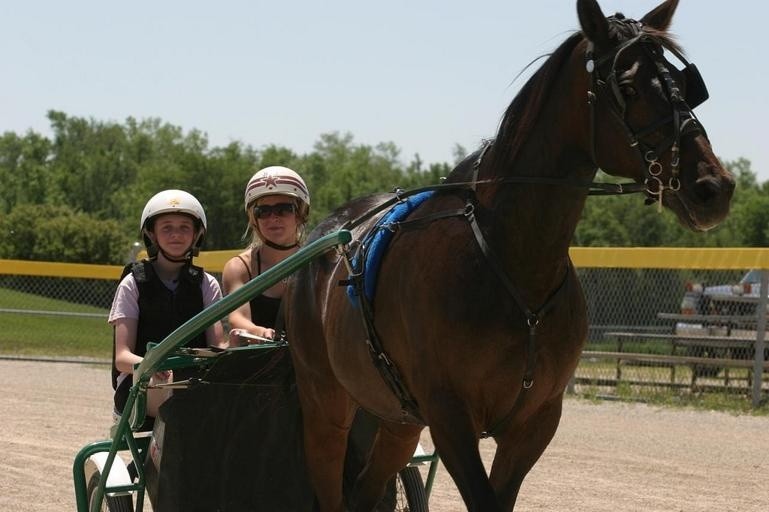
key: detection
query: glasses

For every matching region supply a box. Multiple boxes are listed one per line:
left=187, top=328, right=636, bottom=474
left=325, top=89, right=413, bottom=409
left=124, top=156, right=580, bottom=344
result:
left=252, top=202, right=295, bottom=219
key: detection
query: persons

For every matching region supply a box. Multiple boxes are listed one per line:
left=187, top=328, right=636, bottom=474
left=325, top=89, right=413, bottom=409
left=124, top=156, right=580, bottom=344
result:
left=221, top=165, right=311, bottom=344
left=107, top=189, right=225, bottom=418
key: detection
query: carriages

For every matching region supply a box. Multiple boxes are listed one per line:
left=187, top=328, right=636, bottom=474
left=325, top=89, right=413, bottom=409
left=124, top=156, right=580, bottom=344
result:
left=73, top=2, right=736, bottom=511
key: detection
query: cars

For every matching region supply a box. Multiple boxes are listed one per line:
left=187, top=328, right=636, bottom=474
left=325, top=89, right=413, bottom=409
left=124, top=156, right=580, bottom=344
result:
left=676, top=269, right=769, bottom=376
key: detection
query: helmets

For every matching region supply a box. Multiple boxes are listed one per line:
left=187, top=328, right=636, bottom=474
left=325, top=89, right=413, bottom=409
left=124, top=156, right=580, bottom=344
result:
left=140, top=189, right=208, bottom=246
left=245, top=165, right=311, bottom=211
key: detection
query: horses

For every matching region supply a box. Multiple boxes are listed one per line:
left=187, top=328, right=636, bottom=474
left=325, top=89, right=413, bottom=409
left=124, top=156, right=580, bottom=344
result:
left=283, top=0, right=737, bottom=512
